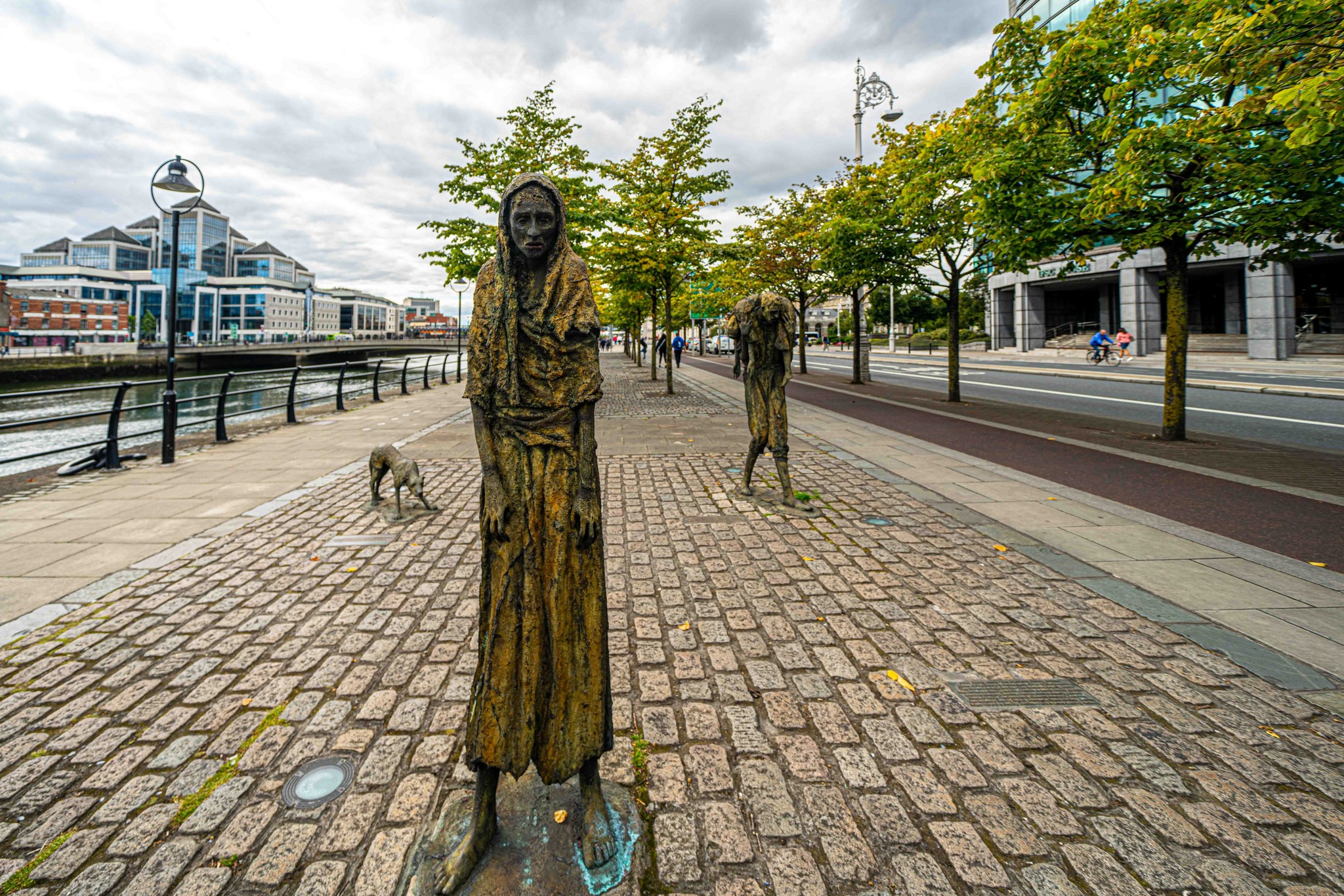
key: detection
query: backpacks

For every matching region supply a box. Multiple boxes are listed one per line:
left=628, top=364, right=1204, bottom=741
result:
left=675, top=338, right=683, bottom=349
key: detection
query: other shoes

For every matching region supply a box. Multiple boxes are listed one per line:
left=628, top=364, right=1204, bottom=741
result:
left=1126, top=357, right=1133, bottom=361
left=677, top=364, right=680, bottom=368
left=1091, top=359, right=1099, bottom=362
left=665, top=365, right=666, bottom=368
left=659, top=365, right=661, bottom=367
left=1117, top=358, right=1124, bottom=361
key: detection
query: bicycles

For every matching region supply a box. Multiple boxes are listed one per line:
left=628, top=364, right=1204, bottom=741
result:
left=1086, top=342, right=1121, bottom=367
left=822, top=342, right=829, bottom=352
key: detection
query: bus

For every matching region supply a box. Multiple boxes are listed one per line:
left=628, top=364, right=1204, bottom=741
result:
left=795, top=332, right=819, bottom=345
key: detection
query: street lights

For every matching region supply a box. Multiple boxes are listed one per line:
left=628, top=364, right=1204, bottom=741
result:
left=150, top=155, right=205, bottom=463
left=853, top=56, right=904, bottom=382
left=450, top=273, right=470, bottom=384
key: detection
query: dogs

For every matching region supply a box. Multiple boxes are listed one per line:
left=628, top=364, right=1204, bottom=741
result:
left=368, top=443, right=438, bottom=521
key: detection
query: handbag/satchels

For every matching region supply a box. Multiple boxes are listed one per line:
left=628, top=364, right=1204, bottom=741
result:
left=657, top=347, right=660, bottom=350
left=822, top=343, right=825, bottom=349
left=1129, top=336, right=1134, bottom=341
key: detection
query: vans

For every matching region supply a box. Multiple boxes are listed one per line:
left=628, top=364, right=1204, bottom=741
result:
left=708, top=335, right=735, bottom=355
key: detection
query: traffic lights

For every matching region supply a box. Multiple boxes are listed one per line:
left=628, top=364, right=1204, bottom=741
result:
left=674, top=330, right=677, bottom=333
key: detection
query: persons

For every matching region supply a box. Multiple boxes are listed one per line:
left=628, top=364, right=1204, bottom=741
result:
left=1090, top=329, right=1117, bottom=362
left=430, top=171, right=617, bottom=896
left=598, top=333, right=633, bottom=352
left=1116, top=328, right=1135, bottom=362
left=656, top=334, right=667, bottom=368
left=671, top=333, right=686, bottom=368
left=822, top=333, right=830, bottom=352
left=804, top=334, right=809, bottom=346
left=726, top=293, right=812, bottom=511
left=636, top=338, right=647, bottom=359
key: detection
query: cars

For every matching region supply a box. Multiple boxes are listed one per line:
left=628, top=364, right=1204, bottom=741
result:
left=685, top=339, right=711, bottom=352
